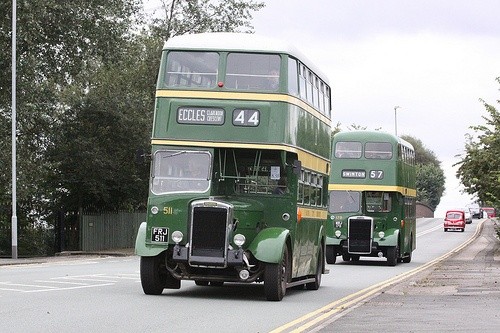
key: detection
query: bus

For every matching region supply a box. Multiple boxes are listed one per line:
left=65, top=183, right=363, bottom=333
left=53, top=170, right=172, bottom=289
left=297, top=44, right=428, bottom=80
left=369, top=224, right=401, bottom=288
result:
left=325, top=131, right=416, bottom=265
left=136, top=32, right=332, bottom=302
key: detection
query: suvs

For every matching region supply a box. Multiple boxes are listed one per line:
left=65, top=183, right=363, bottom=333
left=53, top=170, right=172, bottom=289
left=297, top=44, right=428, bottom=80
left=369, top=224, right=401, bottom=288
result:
left=443, top=211, right=465, bottom=232
left=468, top=205, right=483, bottom=219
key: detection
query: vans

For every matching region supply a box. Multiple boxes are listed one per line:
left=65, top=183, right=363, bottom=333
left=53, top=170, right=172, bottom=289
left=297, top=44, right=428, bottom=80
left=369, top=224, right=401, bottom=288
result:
left=481, top=208, right=496, bottom=219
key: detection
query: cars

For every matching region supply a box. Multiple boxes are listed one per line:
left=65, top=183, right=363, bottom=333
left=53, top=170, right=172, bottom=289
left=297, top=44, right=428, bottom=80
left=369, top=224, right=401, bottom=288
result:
left=454, top=207, right=472, bottom=224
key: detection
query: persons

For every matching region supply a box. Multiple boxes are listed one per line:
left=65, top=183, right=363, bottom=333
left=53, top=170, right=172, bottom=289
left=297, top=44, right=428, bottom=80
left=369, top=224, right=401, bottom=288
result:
left=273, top=177, right=289, bottom=196
left=261, top=69, right=280, bottom=91
